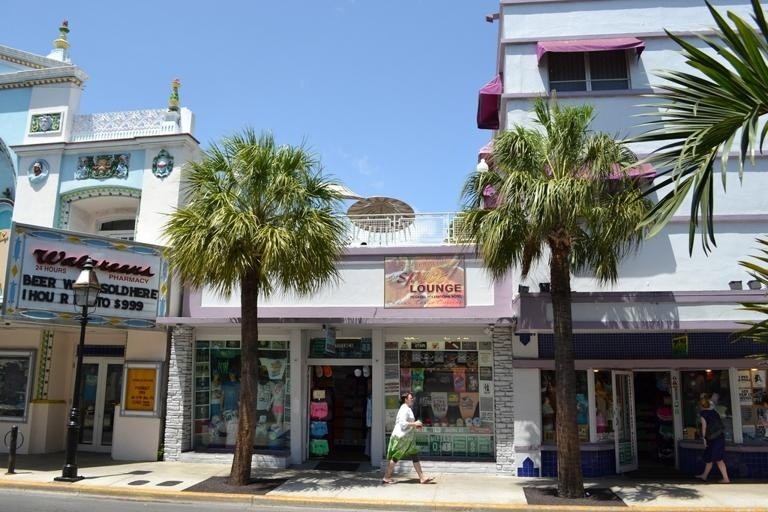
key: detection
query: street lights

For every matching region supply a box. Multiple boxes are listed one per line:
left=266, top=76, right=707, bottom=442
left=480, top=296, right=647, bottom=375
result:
left=54, top=256, right=103, bottom=483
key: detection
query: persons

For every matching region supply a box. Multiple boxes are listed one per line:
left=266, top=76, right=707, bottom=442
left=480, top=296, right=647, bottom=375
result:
left=694, top=397, right=730, bottom=484
left=759, top=397, right=768, bottom=440
left=380, top=391, right=434, bottom=484
left=211, top=366, right=284, bottom=435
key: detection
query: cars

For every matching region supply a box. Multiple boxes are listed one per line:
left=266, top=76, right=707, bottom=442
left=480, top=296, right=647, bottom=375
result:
left=83, top=405, right=111, bottom=430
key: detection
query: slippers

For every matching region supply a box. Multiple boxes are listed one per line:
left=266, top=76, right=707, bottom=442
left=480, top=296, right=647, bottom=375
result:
left=420, top=476, right=435, bottom=484
left=382, top=478, right=398, bottom=484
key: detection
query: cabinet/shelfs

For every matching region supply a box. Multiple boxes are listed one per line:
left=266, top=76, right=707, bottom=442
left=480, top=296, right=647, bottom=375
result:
left=194, top=340, right=211, bottom=422
left=210, top=340, right=290, bottom=350
left=283, top=356, right=290, bottom=425
